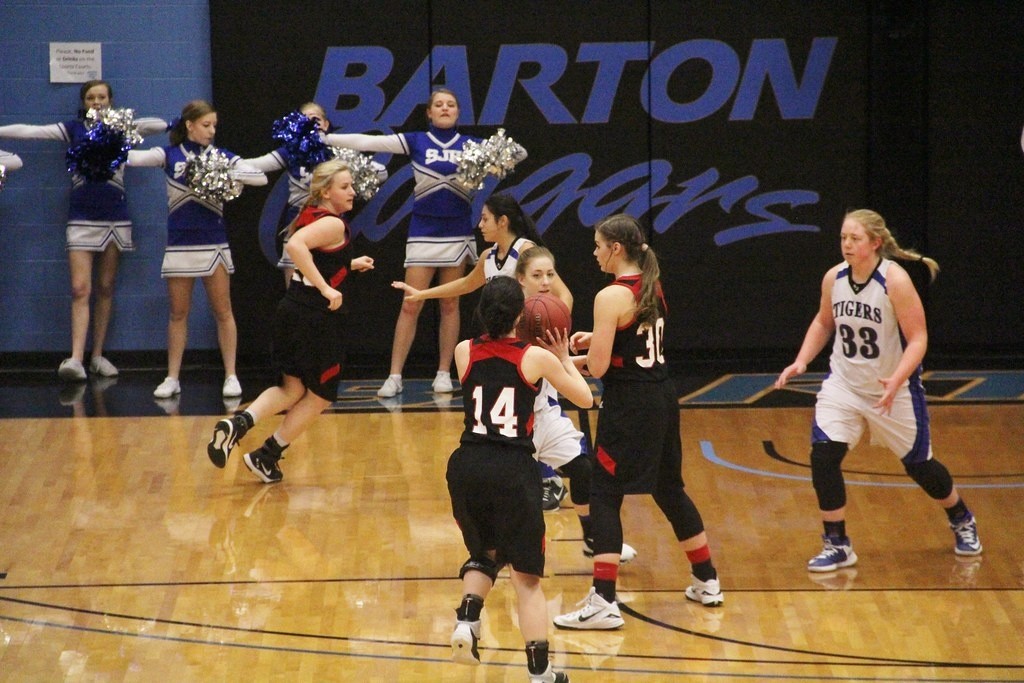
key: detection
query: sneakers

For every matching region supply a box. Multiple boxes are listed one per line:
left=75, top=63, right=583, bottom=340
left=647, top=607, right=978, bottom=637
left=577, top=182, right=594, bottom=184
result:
left=432, top=370, right=453, bottom=392
left=378, top=375, right=403, bottom=397
left=527, top=662, right=569, bottom=683
left=453, top=618, right=481, bottom=664
left=154, top=378, right=181, bottom=397
left=223, top=374, right=242, bottom=397
left=808, top=534, right=858, bottom=571
left=686, top=569, right=724, bottom=607
left=244, top=436, right=283, bottom=483
left=58, top=359, right=87, bottom=381
left=950, top=513, right=982, bottom=555
left=583, top=537, right=637, bottom=565
left=207, top=419, right=241, bottom=468
left=89, top=357, right=119, bottom=377
left=542, top=474, right=569, bottom=512
left=554, top=587, right=625, bottom=630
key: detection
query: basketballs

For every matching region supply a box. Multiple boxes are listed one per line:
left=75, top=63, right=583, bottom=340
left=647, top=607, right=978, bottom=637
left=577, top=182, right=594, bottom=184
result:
left=517, top=294, right=572, bottom=347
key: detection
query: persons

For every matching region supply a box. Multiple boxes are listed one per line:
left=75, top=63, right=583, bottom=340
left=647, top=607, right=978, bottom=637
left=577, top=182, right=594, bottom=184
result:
left=124, top=101, right=268, bottom=398
left=446, top=278, right=594, bottom=683
left=306, top=87, right=528, bottom=396
left=392, top=193, right=574, bottom=511
left=510, top=246, right=637, bottom=564
left=774, top=209, right=982, bottom=573
left=240, top=102, right=388, bottom=297
left=208, top=161, right=375, bottom=483
left=553, top=214, right=725, bottom=631
left=0, top=81, right=169, bottom=381
